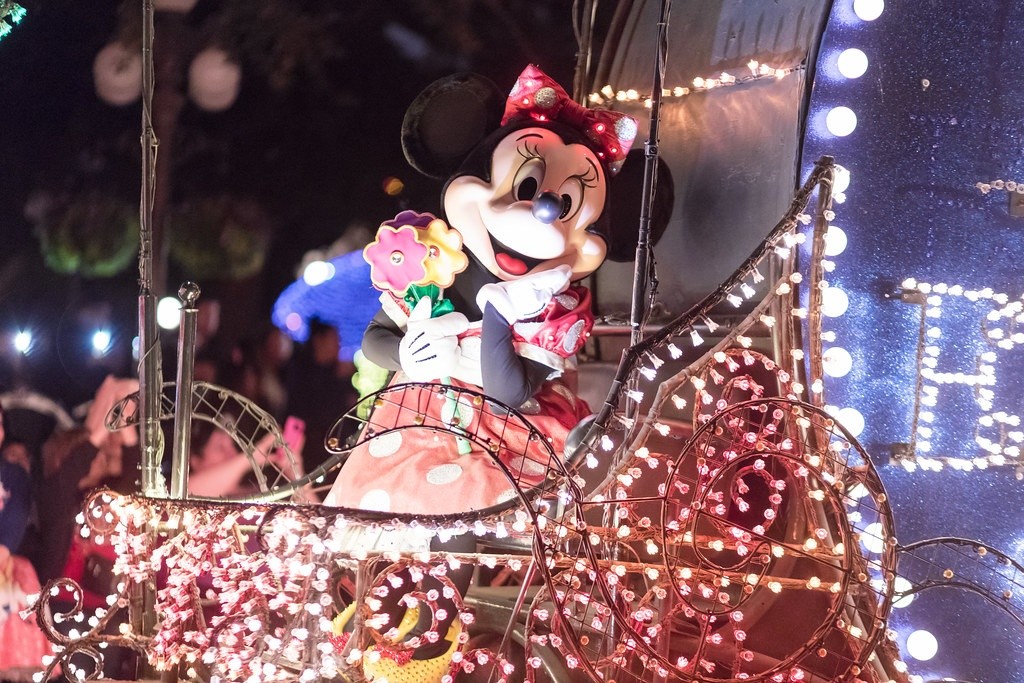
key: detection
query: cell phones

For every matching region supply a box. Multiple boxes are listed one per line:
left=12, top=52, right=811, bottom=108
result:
left=277, top=416, right=305, bottom=461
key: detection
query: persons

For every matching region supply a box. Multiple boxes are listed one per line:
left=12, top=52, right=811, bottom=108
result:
left=0, top=298, right=355, bottom=683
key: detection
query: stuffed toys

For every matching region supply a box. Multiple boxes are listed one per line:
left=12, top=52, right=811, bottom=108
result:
left=320, top=61, right=676, bottom=683
